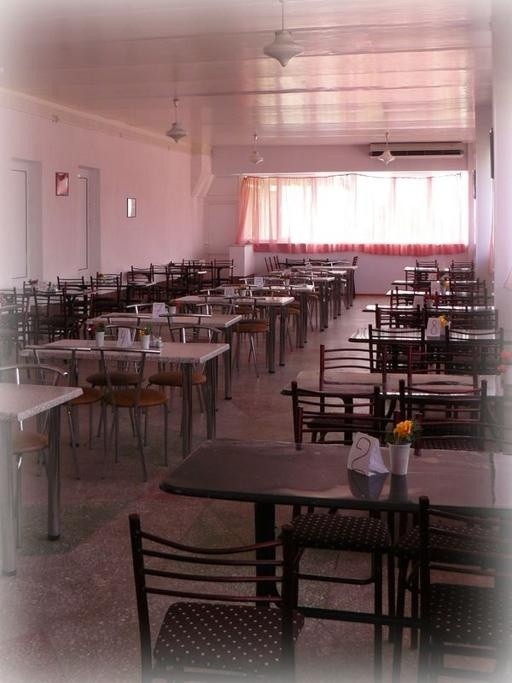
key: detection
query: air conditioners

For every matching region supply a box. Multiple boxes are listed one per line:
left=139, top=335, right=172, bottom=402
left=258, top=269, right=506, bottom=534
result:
left=369, top=142, right=464, bottom=160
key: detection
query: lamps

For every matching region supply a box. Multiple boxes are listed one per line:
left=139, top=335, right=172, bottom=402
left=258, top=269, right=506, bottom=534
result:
left=165, top=96, right=188, bottom=142
left=264, top=0, right=304, bottom=69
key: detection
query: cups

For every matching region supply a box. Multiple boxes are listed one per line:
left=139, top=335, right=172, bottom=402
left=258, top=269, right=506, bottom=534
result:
left=94, top=331, right=105, bottom=346
left=440, top=321, right=451, bottom=334
left=441, top=285, right=446, bottom=293
left=387, top=441, right=412, bottom=474
left=240, top=288, right=246, bottom=296
left=140, top=335, right=150, bottom=348
left=169, top=306, right=176, bottom=314
